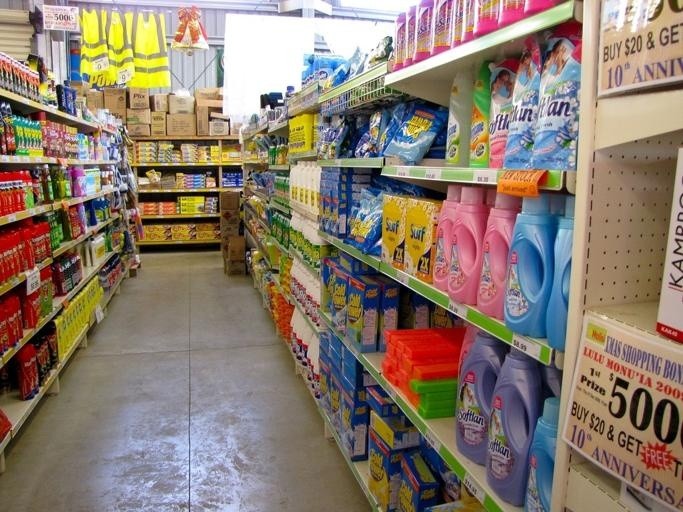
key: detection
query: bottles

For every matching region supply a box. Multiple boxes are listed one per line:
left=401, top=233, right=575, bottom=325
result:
left=288, top=214, right=323, bottom=276
left=289, top=255, right=323, bottom=328
left=0, top=165, right=86, bottom=399
left=288, top=111, right=319, bottom=157
left=289, top=160, right=322, bottom=224
left=286, top=308, right=321, bottom=395
left=272, top=144, right=290, bottom=252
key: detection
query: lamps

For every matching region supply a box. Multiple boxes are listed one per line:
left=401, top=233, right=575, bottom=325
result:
left=171, top=4, right=210, bottom=56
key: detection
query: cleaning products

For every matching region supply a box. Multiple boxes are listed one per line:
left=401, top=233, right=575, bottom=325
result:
left=487, top=58, right=520, bottom=168
left=474, top=187, right=521, bottom=321
left=456, top=329, right=506, bottom=467
left=485, top=346, right=543, bottom=508
left=445, top=71, right=475, bottom=168
left=544, top=197, right=576, bottom=352
left=468, top=58, right=491, bottom=168
left=503, top=193, right=554, bottom=338
left=474, top=0, right=559, bottom=38
left=502, top=35, right=543, bottom=169
left=523, top=397, right=560, bottom=511
left=529, top=19, right=581, bottom=169
left=432, top=183, right=461, bottom=293
left=393, top=1, right=475, bottom=71
left=446, top=187, right=488, bottom=306
left=285, top=160, right=321, bottom=402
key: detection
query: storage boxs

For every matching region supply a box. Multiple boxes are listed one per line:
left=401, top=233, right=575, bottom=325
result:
left=104, top=88, right=126, bottom=125
left=220, top=225, right=246, bottom=278
left=129, top=88, right=150, bottom=109
left=150, top=88, right=230, bottom=135
left=127, top=125, right=150, bottom=136
left=127, top=108, right=151, bottom=125
left=220, top=191, right=240, bottom=210
left=221, top=210, right=240, bottom=225
left=87, top=91, right=104, bottom=109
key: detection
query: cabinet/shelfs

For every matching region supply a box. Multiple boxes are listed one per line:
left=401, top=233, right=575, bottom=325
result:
left=242, top=0, right=581, bottom=512
left=0, top=88, right=141, bottom=473
left=552, top=0, right=683, bottom=512
left=129, top=134, right=241, bottom=245
